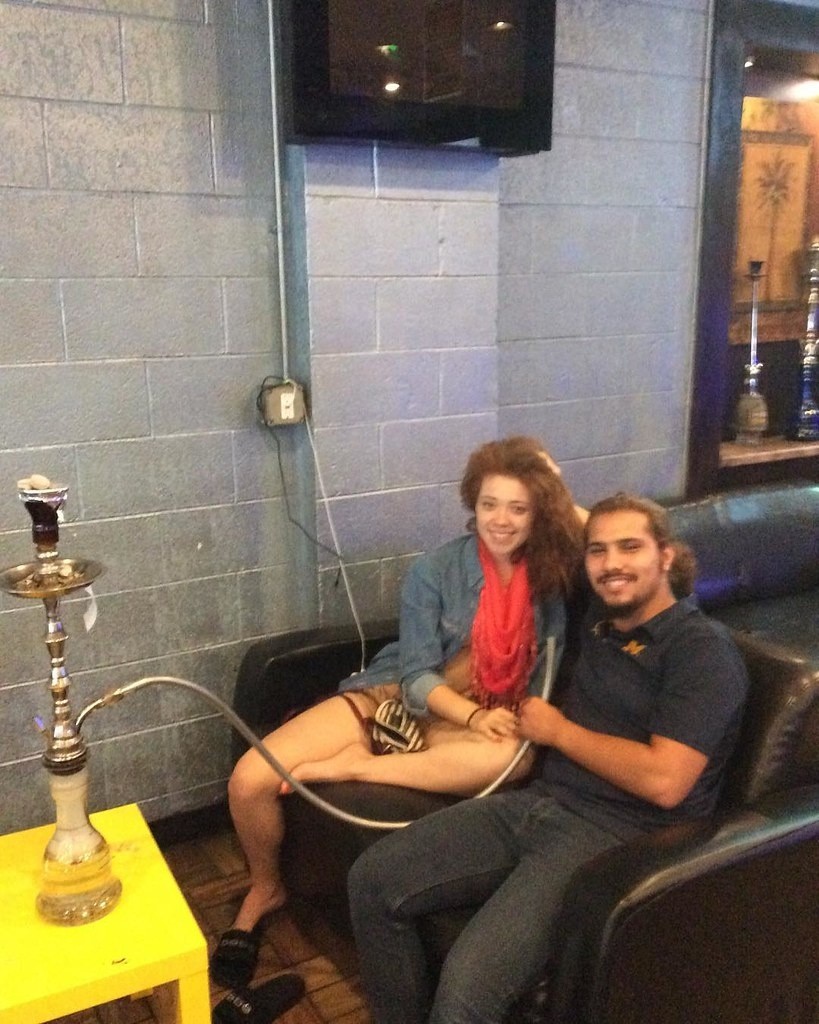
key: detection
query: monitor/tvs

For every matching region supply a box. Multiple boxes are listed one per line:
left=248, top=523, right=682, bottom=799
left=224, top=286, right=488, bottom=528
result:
left=289, top=0, right=558, bottom=154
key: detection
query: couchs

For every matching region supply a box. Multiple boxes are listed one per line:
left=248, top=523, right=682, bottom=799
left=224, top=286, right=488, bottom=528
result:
left=231, top=478, right=819, bottom=1024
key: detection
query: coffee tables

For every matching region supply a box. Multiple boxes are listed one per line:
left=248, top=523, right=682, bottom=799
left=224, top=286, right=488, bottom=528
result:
left=0, top=803, right=215, bottom=1024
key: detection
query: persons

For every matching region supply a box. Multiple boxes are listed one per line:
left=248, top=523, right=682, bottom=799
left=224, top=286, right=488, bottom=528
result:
left=348, top=490, right=748, bottom=1024
left=228, top=436, right=591, bottom=935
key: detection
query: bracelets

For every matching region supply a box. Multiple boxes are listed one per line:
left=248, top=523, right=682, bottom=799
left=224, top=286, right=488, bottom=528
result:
left=467, top=707, right=485, bottom=730
